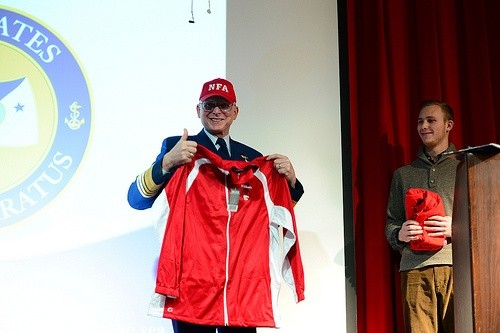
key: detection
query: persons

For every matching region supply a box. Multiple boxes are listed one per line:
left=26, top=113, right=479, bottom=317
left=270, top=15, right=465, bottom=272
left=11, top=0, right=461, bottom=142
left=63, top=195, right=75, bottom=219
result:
left=127, top=78, right=305, bottom=333
left=385, top=100, right=464, bottom=333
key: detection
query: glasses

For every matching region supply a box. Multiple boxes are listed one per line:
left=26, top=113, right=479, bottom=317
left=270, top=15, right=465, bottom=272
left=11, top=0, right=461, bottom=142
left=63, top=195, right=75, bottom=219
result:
left=199, top=103, right=236, bottom=112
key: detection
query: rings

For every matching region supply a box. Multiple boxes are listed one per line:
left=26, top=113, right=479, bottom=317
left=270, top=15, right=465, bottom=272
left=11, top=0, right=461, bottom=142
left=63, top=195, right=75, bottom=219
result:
left=189, top=153, right=193, bottom=158
left=279, top=164, right=281, bottom=169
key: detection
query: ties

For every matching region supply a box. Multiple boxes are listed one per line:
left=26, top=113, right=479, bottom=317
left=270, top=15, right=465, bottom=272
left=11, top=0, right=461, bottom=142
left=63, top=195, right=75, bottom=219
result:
left=217, top=138, right=231, bottom=163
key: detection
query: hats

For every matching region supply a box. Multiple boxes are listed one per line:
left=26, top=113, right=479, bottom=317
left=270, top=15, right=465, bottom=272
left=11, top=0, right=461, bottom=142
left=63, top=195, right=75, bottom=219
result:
left=199, top=78, right=237, bottom=106
